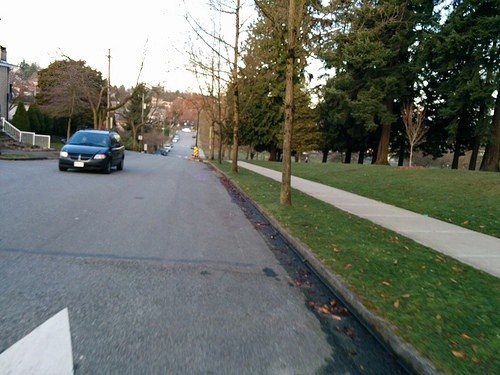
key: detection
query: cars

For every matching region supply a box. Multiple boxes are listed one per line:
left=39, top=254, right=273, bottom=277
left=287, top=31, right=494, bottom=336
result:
left=58, top=129, right=125, bottom=174
left=159, top=125, right=194, bottom=156
